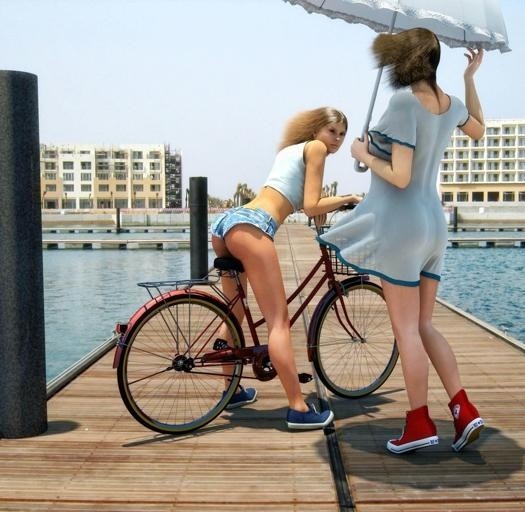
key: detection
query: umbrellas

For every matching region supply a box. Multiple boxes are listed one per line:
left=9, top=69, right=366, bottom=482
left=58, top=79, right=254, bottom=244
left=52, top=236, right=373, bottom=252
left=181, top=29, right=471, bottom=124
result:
left=281, top=0, right=511, bottom=172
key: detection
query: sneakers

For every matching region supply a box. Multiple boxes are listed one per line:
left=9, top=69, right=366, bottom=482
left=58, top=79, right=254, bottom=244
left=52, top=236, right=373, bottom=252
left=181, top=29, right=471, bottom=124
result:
left=222, top=387, right=259, bottom=408
left=285, top=402, right=335, bottom=430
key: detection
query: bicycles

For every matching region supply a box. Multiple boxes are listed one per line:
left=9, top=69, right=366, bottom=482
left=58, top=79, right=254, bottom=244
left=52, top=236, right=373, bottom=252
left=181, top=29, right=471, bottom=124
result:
left=112, top=205, right=399, bottom=434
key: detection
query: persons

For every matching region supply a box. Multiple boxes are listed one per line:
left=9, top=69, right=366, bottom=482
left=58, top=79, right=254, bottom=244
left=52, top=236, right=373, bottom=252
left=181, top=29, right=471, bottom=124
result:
left=208, top=104, right=368, bottom=431
left=313, top=26, right=487, bottom=459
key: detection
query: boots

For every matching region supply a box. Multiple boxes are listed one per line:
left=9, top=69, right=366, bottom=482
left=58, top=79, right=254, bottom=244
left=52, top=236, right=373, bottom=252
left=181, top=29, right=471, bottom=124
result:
left=387, top=407, right=437, bottom=455
left=445, top=388, right=486, bottom=454
